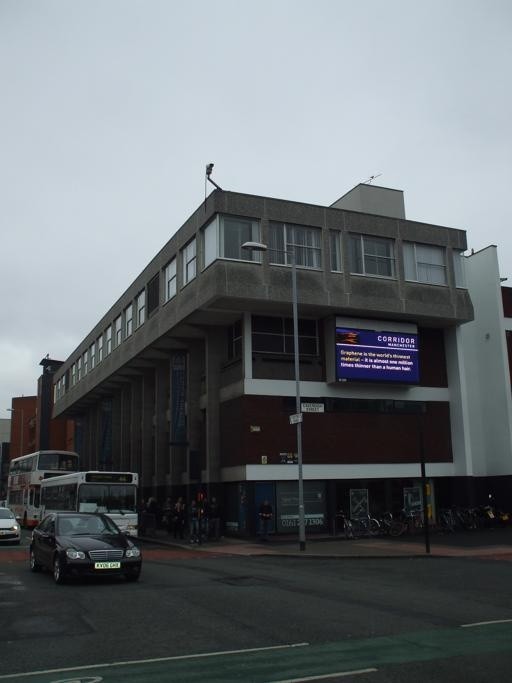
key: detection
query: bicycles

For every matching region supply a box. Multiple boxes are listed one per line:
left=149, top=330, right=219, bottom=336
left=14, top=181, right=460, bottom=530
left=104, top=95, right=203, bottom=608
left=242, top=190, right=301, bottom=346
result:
left=441, top=507, right=476, bottom=535
left=337, top=509, right=409, bottom=539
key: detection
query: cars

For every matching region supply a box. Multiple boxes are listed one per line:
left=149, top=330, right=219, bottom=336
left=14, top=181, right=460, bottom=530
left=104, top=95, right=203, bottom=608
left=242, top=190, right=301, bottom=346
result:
left=0, top=507, right=21, bottom=543
left=29, top=512, right=143, bottom=584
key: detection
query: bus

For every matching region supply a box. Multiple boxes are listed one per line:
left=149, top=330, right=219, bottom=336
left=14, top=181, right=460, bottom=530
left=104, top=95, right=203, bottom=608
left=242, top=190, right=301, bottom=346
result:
left=6, top=450, right=80, bottom=527
left=40, top=469, right=139, bottom=538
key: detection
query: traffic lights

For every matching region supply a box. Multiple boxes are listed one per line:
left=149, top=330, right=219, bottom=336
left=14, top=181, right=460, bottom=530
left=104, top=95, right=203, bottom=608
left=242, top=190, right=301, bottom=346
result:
left=195, top=487, right=207, bottom=518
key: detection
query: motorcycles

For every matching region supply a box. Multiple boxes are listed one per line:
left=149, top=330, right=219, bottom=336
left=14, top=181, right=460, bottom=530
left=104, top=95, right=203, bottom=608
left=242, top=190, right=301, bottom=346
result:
left=477, top=492, right=497, bottom=526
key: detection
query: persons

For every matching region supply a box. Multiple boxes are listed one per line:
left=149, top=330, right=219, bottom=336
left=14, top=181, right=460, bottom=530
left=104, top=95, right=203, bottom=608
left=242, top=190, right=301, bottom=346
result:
left=259, top=498, right=273, bottom=515
left=146, top=493, right=222, bottom=547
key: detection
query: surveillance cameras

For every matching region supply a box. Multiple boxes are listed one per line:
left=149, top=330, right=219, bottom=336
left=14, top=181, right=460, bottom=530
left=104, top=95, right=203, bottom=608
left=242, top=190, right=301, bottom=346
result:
left=206, top=163, right=214, bottom=169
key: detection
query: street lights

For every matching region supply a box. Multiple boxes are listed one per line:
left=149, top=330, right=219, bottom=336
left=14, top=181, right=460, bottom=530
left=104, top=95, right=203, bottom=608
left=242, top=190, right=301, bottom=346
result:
left=243, top=241, right=306, bottom=551
